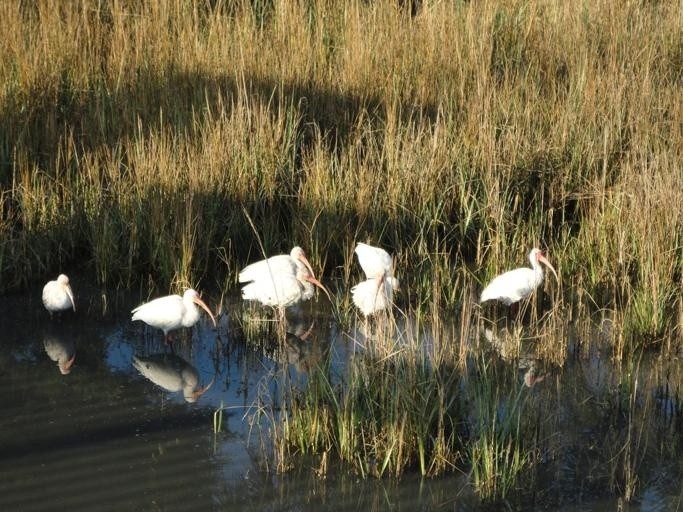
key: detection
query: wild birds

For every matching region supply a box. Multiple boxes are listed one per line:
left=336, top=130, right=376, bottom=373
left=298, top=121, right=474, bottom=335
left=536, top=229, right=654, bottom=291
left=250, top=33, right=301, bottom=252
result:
left=43, top=317, right=76, bottom=375
left=247, top=318, right=329, bottom=373
left=479, top=247, right=558, bottom=317
left=41, top=273, right=77, bottom=316
left=238, top=246, right=331, bottom=319
left=130, top=344, right=215, bottom=404
left=130, top=288, right=217, bottom=345
left=349, top=241, right=400, bottom=332
